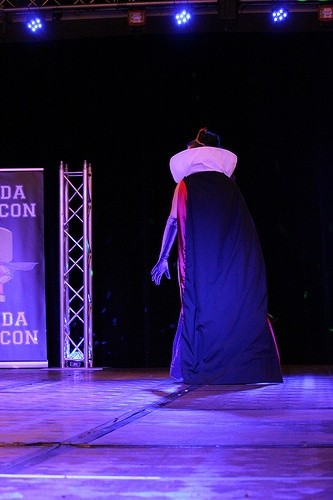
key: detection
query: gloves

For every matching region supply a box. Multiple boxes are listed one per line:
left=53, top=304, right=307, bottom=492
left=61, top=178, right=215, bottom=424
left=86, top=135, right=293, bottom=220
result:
left=150, top=216, right=178, bottom=286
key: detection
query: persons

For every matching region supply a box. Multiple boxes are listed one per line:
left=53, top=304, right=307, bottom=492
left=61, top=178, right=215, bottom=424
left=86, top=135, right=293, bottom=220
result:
left=150, top=126, right=283, bottom=384
